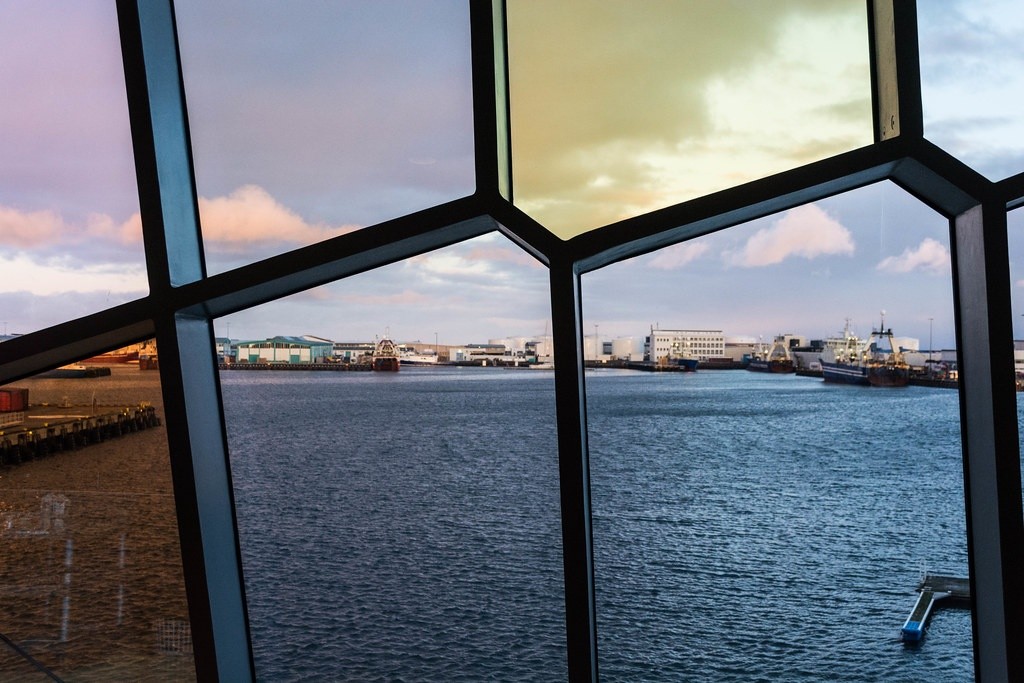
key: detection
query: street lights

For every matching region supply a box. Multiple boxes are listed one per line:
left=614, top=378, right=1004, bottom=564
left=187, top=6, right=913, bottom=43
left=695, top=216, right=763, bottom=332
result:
left=928, top=318, right=934, bottom=372
left=434, top=332, right=438, bottom=352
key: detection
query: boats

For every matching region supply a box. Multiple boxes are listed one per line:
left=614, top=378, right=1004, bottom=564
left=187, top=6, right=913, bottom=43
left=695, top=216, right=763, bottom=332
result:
left=740, top=331, right=797, bottom=374
left=371, top=339, right=400, bottom=371
left=816, top=317, right=916, bottom=387
